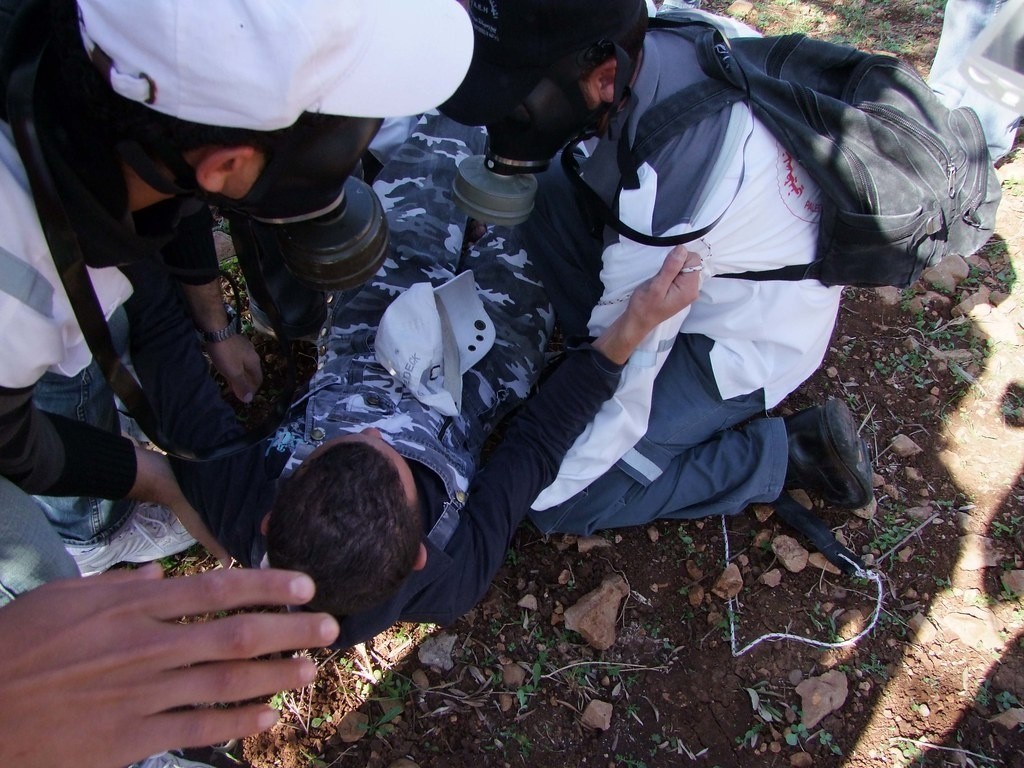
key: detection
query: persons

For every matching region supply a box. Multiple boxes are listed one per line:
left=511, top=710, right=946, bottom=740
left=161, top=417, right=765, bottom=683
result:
left=929, top=0, right=1024, bottom=165
left=0, top=0, right=871, bottom=768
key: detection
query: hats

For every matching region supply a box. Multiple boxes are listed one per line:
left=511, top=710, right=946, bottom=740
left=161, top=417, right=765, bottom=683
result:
left=70, top=0, right=476, bottom=134
left=437, top=0, right=645, bottom=128
left=376, top=269, right=497, bottom=416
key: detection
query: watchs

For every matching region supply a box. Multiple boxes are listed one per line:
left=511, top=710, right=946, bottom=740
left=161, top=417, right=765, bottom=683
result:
left=204, top=302, right=237, bottom=343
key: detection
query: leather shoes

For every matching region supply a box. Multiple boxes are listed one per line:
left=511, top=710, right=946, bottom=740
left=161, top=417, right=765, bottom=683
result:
left=785, top=399, right=873, bottom=511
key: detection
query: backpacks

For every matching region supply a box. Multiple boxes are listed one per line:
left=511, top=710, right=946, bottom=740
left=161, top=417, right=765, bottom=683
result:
left=626, top=14, right=1001, bottom=290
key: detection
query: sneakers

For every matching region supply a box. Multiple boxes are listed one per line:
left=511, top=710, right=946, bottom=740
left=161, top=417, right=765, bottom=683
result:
left=139, top=748, right=217, bottom=768
left=65, top=499, right=198, bottom=579
